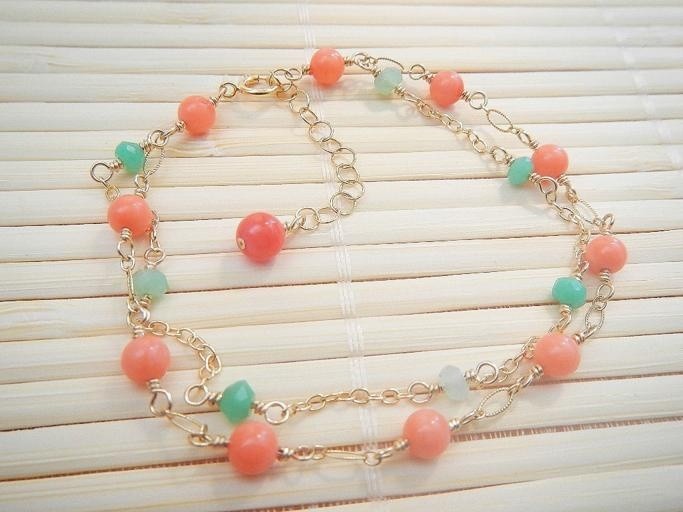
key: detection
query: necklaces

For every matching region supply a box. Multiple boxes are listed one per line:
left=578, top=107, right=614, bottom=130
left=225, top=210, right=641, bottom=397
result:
left=91, top=46, right=627, bottom=478
